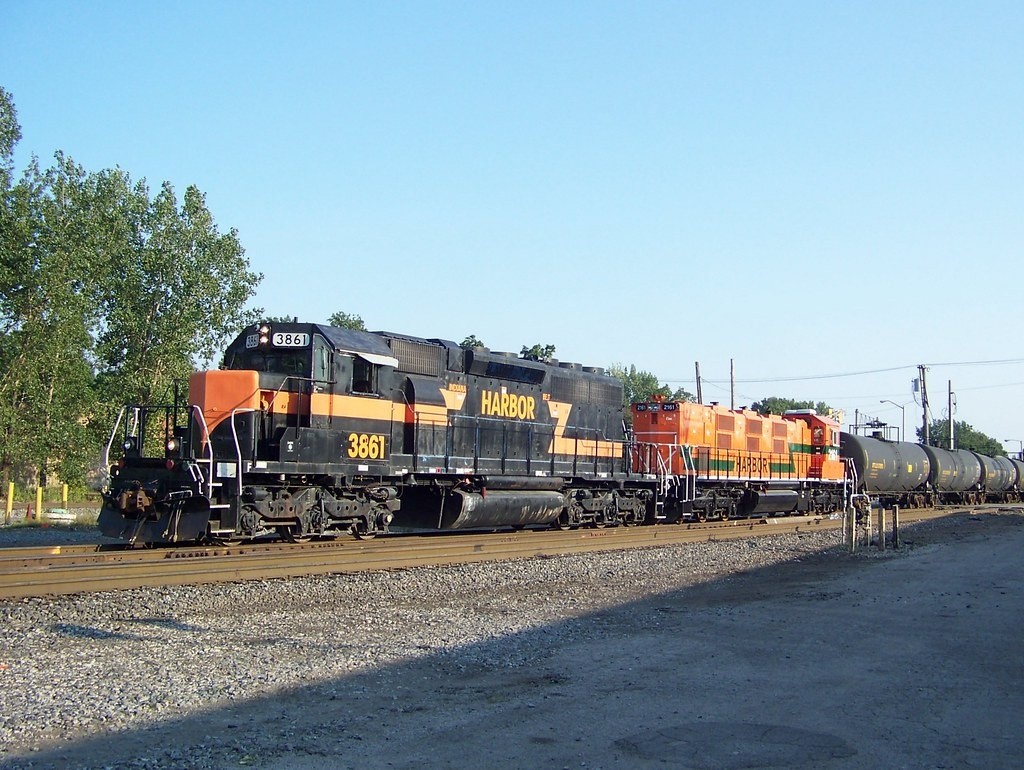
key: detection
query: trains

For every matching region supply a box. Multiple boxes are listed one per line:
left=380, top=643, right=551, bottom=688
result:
left=95, top=316, right=1024, bottom=548
